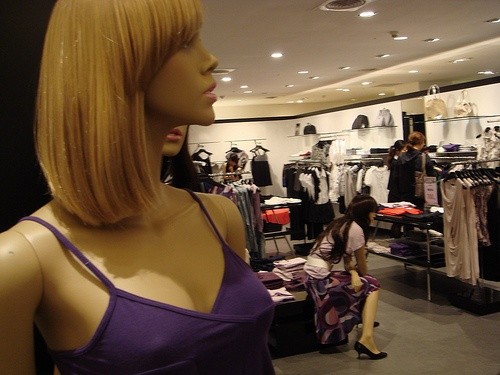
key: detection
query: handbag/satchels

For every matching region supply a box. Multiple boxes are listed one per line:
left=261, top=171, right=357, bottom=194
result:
left=352, top=115, right=369, bottom=129
left=425, top=83, right=448, bottom=122
left=454, top=89, right=472, bottom=118
left=414, top=153, right=428, bottom=197
left=304, top=123, right=317, bottom=134
left=375, top=109, right=394, bottom=127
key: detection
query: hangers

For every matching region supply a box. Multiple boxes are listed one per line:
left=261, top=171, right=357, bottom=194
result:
left=443, top=159, right=500, bottom=187
left=195, top=138, right=382, bottom=189
left=476, top=121, right=500, bottom=139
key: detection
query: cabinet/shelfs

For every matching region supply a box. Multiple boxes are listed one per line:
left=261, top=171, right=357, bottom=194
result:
left=259, top=203, right=297, bottom=257
left=365, top=212, right=446, bottom=300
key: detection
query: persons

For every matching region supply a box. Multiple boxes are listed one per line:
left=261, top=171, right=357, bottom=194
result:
left=302, top=193, right=389, bottom=361
left=386, top=131, right=440, bottom=238
left=222, top=153, right=242, bottom=181
left=0, top=0, right=277, bottom=375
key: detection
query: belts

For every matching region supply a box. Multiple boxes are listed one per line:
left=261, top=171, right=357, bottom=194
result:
left=306, top=256, right=334, bottom=271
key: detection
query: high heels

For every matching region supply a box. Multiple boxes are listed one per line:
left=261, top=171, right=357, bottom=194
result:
left=354, top=342, right=388, bottom=360
left=354, top=319, right=380, bottom=328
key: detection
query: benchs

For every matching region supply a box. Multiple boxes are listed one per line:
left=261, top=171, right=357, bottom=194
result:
left=268, top=288, right=316, bottom=362
